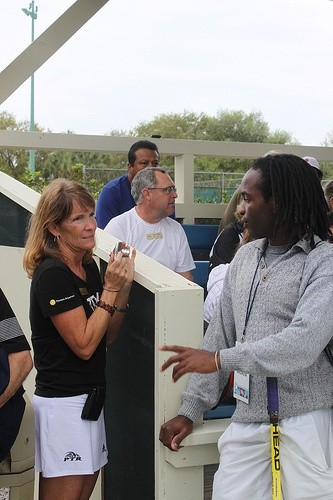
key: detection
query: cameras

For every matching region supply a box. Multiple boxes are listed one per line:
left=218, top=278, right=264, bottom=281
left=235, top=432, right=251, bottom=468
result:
left=113, top=242, right=130, bottom=260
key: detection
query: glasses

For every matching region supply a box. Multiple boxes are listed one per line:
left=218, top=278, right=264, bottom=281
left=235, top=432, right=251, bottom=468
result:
left=148, top=186, right=177, bottom=193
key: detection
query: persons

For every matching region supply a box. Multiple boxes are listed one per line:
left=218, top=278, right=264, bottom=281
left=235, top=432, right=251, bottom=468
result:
left=96, top=140, right=160, bottom=230
left=159, top=154, right=333, bottom=500
left=0, top=288, right=33, bottom=463
left=103, top=167, right=196, bottom=281
left=23, top=178, right=136, bottom=500
left=204, top=150, right=333, bottom=329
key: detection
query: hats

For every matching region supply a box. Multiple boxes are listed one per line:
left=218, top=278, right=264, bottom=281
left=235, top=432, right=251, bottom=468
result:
left=303, top=157, right=323, bottom=180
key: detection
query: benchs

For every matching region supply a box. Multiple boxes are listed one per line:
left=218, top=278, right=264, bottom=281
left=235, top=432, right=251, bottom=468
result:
left=179, top=225, right=236, bottom=420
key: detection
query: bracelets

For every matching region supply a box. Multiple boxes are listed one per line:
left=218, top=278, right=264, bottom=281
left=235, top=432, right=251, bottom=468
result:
left=115, top=304, right=130, bottom=313
left=97, top=300, right=115, bottom=316
left=215, top=351, right=220, bottom=371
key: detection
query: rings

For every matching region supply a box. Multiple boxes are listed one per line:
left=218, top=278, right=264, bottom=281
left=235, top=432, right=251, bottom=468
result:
left=123, top=267, right=128, bottom=272
